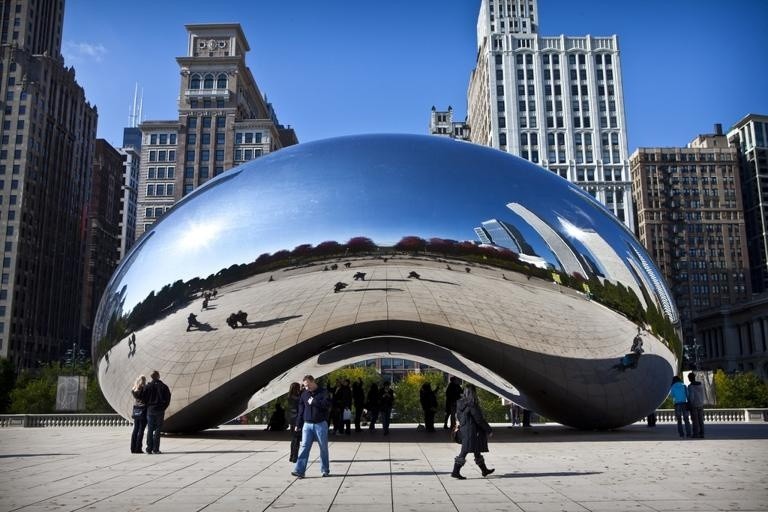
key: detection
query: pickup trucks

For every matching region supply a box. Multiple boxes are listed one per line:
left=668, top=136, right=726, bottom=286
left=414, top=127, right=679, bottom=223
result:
left=641, top=321, right=653, bottom=334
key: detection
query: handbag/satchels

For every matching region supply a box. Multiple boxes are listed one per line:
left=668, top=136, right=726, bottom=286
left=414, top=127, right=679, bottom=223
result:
left=454, top=425, right=462, bottom=443
left=132, top=406, right=145, bottom=418
left=343, top=409, right=351, bottom=421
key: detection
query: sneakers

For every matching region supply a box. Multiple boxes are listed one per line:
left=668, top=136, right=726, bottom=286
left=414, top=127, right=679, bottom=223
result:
left=323, top=472, right=328, bottom=476
left=291, top=471, right=305, bottom=478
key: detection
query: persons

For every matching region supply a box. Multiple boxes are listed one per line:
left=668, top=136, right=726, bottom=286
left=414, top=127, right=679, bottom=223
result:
left=226, top=313, right=239, bottom=329
left=648, top=413, right=657, bottom=429
left=200, top=288, right=217, bottom=312
left=669, top=376, right=691, bottom=440
left=407, top=271, right=420, bottom=279
left=688, top=372, right=706, bottom=438
left=236, top=311, right=249, bottom=326
left=128, top=338, right=133, bottom=351
left=131, top=375, right=147, bottom=453
left=608, top=327, right=644, bottom=376
left=131, top=370, right=171, bottom=454
left=263, top=375, right=532, bottom=479
left=186, top=313, right=200, bottom=332
left=104, top=351, right=109, bottom=363
left=132, top=332, right=136, bottom=349
left=465, top=267, right=471, bottom=273
left=322, top=262, right=367, bottom=294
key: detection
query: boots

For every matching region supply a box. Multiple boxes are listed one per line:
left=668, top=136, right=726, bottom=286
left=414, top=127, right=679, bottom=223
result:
left=451, top=456, right=466, bottom=479
left=475, top=456, right=495, bottom=476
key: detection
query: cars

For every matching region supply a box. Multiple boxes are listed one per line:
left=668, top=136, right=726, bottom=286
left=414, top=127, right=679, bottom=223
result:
left=657, top=334, right=669, bottom=347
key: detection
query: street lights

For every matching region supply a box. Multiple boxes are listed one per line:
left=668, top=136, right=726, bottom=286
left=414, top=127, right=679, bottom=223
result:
left=287, top=256, right=291, bottom=266
left=344, top=247, right=349, bottom=257
left=64, top=342, right=86, bottom=376
